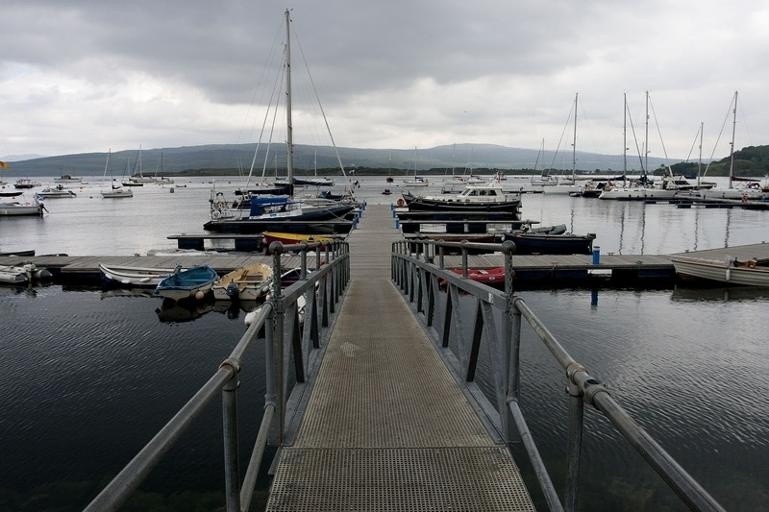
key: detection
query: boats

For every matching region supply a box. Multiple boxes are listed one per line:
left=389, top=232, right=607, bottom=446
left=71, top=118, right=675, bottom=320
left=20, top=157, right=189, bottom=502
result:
left=392, top=170, right=598, bottom=256
left=401, top=136, right=547, bottom=196
left=524, top=83, right=769, bottom=207
left=96, top=247, right=320, bottom=333
left=434, top=262, right=518, bottom=288
left=263, top=229, right=350, bottom=249
left=0, top=263, right=55, bottom=285
left=200, top=4, right=368, bottom=235
left=0, top=171, right=87, bottom=219
left=669, top=253, right=769, bottom=292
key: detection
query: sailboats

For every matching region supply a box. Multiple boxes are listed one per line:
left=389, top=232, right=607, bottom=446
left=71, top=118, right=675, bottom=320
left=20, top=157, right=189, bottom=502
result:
left=96, top=142, right=175, bottom=199
